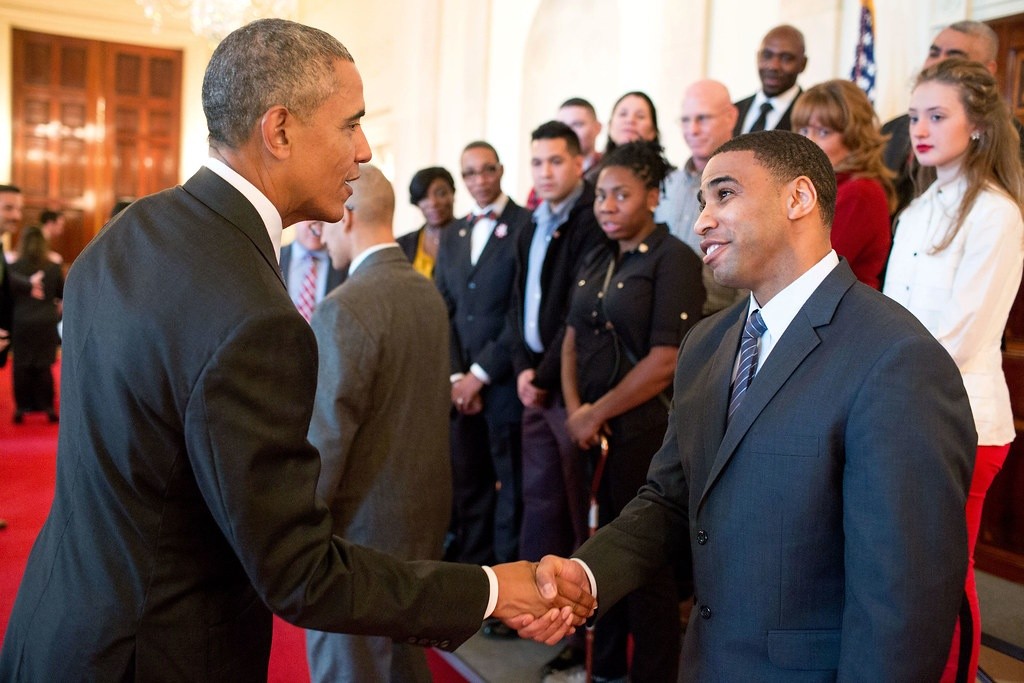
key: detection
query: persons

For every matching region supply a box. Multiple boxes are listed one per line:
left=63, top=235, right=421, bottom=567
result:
left=652, top=78, right=739, bottom=256
left=394, top=166, right=456, bottom=282
left=0, top=18, right=596, bottom=683
left=281, top=219, right=351, bottom=323
left=731, top=26, right=807, bottom=137
left=505, top=130, right=978, bottom=683
left=434, top=141, right=536, bottom=567
left=482, top=119, right=610, bottom=680
left=878, top=19, right=1024, bottom=683
left=560, top=137, right=707, bottom=683
left=0, top=184, right=134, bottom=424
left=604, top=91, right=678, bottom=183
left=790, top=80, right=899, bottom=291
left=525, top=96, right=600, bottom=213
left=306, top=162, right=450, bottom=683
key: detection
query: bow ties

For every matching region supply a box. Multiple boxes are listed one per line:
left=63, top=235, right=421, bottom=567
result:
left=466, top=212, right=498, bottom=228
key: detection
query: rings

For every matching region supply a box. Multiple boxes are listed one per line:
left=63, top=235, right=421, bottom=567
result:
left=456, top=396, right=465, bottom=405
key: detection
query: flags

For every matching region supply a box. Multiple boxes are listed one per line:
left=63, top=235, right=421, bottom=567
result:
left=844, top=0, right=876, bottom=111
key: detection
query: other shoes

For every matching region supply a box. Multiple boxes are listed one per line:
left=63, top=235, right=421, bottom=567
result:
left=544, top=645, right=587, bottom=677
left=482, top=617, right=523, bottom=639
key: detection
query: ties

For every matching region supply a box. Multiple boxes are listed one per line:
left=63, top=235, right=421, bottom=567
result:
left=727, top=309, right=766, bottom=429
left=298, top=253, right=319, bottom=324
left=751, top=102, right=774, bottom=135
